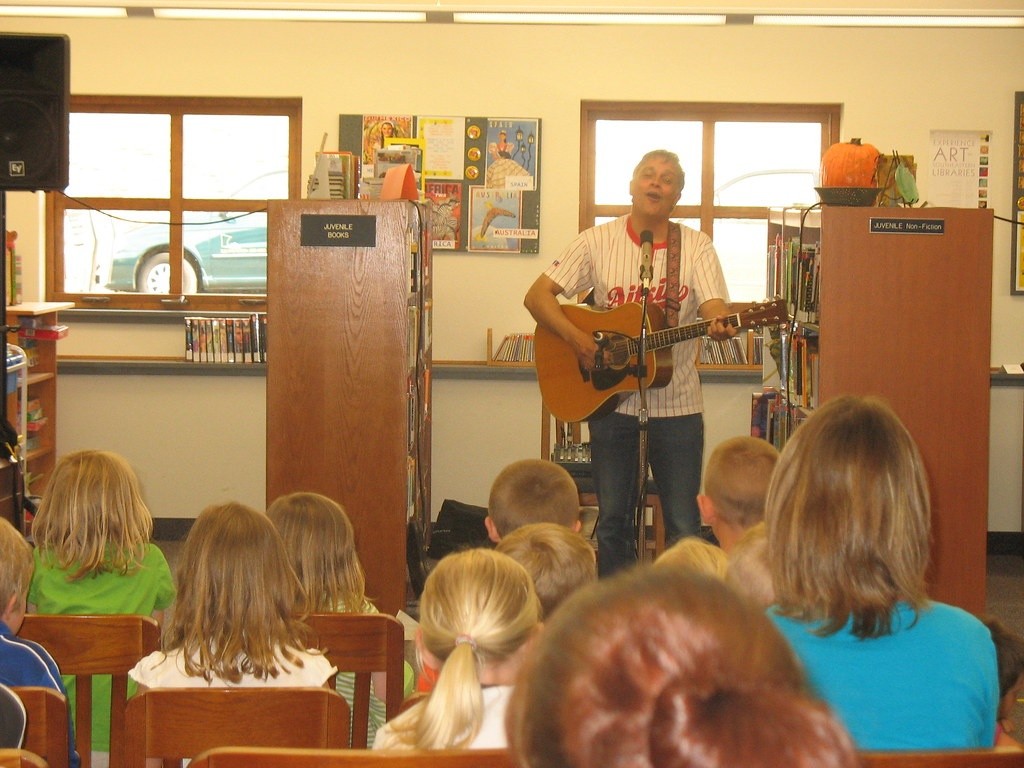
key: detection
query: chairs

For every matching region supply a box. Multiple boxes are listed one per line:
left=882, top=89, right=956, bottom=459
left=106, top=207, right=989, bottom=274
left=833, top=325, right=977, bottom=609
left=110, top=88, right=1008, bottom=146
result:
left=542, top=401, right=665, bottom=561
left=0, top=615, right=517, bottom=768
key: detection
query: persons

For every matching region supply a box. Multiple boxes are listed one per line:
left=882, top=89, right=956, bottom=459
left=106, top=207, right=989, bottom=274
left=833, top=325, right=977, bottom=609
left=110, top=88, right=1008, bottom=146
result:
left=652, top=434, right=781, bottom=613
left=524, top=150, right=738, bottom=581
left=759, top=396, right=999, bottom=751
left=371, top=459, right=598, bottom=750
left=128, top=491, right=414, bottom=768
left=503, top=562, right=864, bottom=768
left=982, top=614, right=1024, bottom=749
left=0, top=451, right=177, bottom=768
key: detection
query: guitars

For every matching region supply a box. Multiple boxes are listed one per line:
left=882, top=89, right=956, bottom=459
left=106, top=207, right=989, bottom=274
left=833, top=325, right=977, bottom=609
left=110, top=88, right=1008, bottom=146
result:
left=532, top=295, right=793, bottom=425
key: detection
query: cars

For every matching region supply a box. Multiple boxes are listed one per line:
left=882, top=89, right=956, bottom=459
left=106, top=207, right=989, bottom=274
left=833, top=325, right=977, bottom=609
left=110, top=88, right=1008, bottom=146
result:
left=106, top=170, right=289, bottom=295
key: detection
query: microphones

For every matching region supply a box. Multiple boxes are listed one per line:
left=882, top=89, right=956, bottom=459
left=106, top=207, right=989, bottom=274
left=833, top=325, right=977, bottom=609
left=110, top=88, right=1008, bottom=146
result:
left=640, top=230, right=654, bottom=296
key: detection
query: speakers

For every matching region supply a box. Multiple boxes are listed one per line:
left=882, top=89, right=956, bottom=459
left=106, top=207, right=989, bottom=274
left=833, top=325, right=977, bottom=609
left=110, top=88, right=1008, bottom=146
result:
left=0, top=35, right=69, bottom=193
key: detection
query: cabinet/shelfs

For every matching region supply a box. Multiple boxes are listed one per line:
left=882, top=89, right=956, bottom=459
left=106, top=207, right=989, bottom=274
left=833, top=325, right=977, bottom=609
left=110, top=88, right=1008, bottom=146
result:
left=266, top=198, right=434, bottom=615
left=766, top=207, right=997, bottom=615
left=0, top=302, right=76, bottom=544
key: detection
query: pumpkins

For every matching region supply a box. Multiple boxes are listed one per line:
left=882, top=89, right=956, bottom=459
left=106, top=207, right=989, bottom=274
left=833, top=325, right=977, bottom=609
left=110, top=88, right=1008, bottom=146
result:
left=819, top=138, right=881, bottom=188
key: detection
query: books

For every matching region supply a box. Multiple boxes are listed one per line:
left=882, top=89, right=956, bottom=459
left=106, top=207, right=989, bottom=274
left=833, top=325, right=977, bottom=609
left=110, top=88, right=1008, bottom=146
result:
left=315, top=151, right=360, bottom=199
left=751, top=234, right=821, bottom=452
left=407, top=224, right=430, bottom=598
left=492, top=329, right=764, bottom=366
left=551, top=441, right=652, bottom=561
left=185, top=312, right=267, bottom=364
left=5, top=244, right=68, bottom=536
